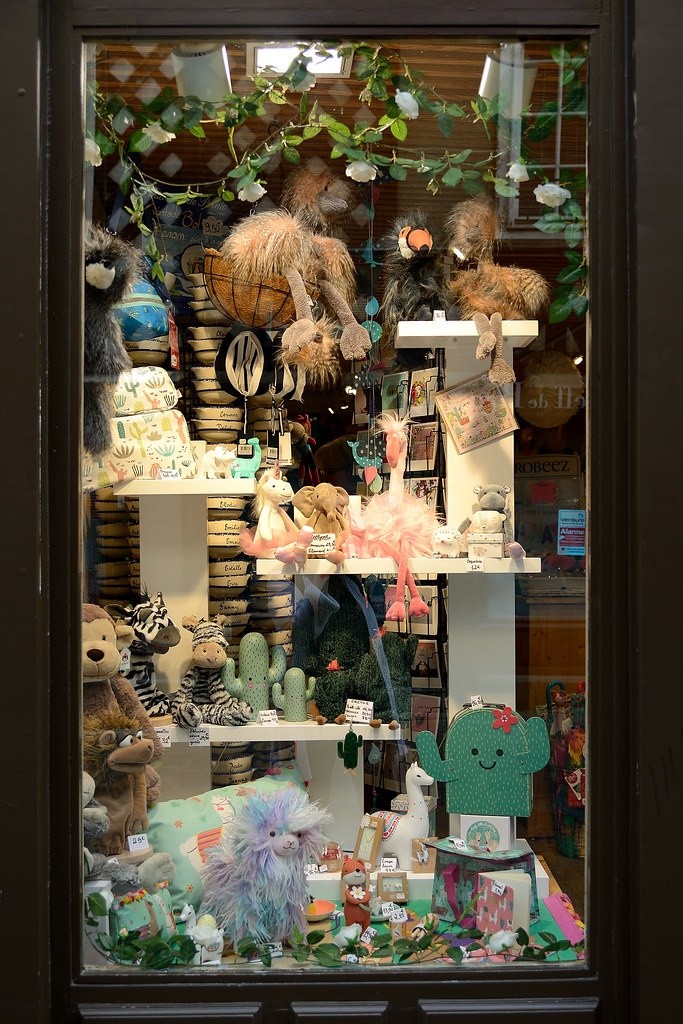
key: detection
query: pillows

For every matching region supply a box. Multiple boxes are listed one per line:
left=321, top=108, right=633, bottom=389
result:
left=112, top=888, right=180, bottom=966
left=143, top=760, right=309, bottom=922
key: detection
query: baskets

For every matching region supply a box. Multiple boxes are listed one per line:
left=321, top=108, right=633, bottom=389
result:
left=546, top=766, right=586, bottom=859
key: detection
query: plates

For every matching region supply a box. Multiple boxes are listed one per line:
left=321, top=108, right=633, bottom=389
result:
left=370, top=903, right=401, bottom=921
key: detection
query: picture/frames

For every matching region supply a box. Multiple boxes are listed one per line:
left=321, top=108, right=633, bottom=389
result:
left=433, top=368, right=521, bottom=453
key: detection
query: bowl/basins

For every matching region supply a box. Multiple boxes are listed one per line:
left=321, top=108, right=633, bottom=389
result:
left=306, top=900, right=336, bottom=921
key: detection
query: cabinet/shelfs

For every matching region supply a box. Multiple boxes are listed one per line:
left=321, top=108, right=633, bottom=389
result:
left=97, top=319, right=544, bottom=909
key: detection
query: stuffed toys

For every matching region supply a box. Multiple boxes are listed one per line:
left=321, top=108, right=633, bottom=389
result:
left=341, top=856, right=372, bottom=938
left=81, top=590, right=255, bottom=896
left=220, top=158, right=371, bottom=389
left=84, top=226, right=135, bottom=459
left=204, top=788, right=332, bottom=955
left=239, top=416, right=526, bottom=622
left=383, top=198, right=550, bottom=387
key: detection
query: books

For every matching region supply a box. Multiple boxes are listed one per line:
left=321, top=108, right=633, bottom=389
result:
left=477, top=869, right=532, bottom=935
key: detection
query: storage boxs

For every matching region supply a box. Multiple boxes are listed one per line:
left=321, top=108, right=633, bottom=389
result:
left=467, top=533, right=506, bottom=559
left=413, top=836, right=438, bottom=874
left=190, top=441, right=208, bottom=481
left=353, top=816, right=385, bottom=872
left=377, top=871, right=409, bottom=903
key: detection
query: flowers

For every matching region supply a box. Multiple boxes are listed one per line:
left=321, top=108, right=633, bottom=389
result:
left=86, top=892, right=585, bottom=970
left=82, top=40, right=593, bottom=325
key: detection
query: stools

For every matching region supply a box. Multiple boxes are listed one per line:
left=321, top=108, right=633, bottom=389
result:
left=84, top=880, right=118, bottom=965
